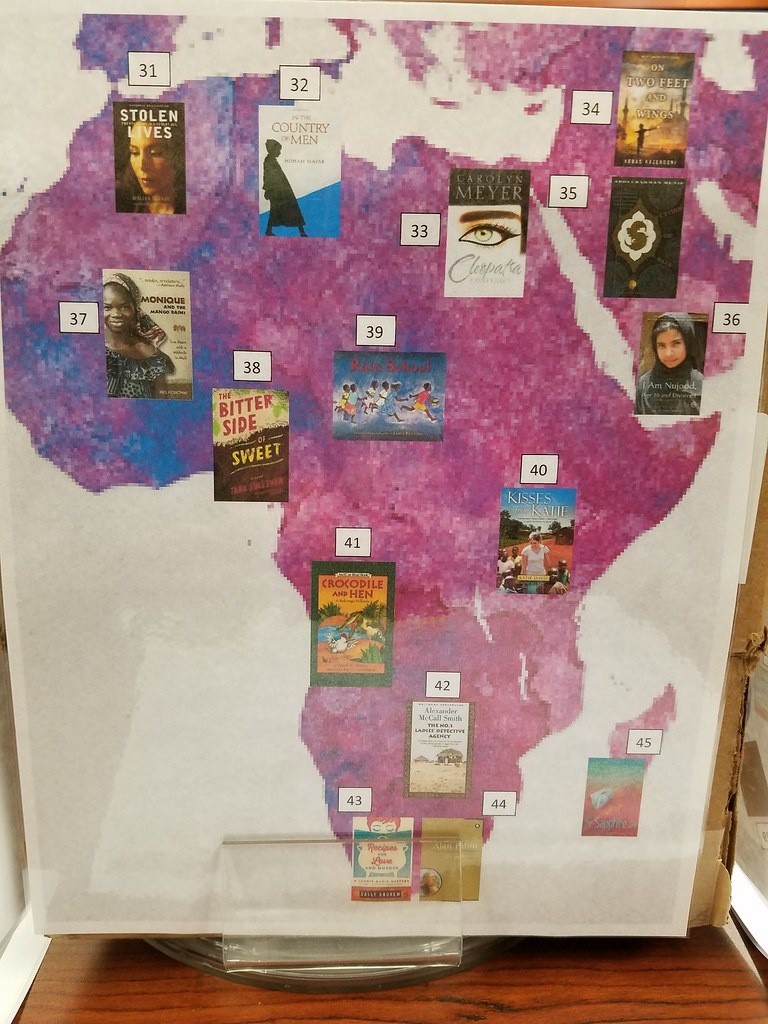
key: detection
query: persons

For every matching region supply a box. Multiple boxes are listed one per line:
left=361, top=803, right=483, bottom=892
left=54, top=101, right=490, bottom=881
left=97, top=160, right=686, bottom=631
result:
left=121, top=111, right=187, bottom=215
left=102, top=273, right=168, bottom=399
left=495, top=531, right=570, bottom=595
left=634, top=311, right=704, bottom=415
